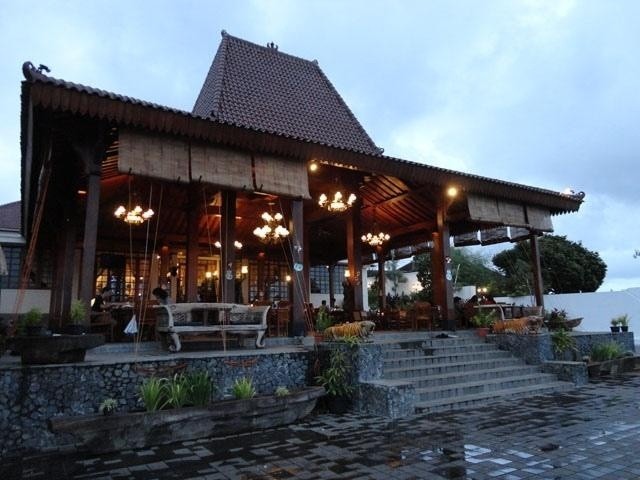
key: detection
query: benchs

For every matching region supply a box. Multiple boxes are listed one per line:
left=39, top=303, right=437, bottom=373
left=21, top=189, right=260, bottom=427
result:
left=152, top=303, right=269, bottom=352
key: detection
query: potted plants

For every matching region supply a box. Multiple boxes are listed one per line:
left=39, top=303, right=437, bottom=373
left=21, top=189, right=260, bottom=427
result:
left=610, top=318, right=621, bottom=332
left=619, top=314, right=631, bottom=332
left=38, top=367, right=325, bottom=446
left=11, top=299, right=105, bottom=365
left=470, top=308, right=497, bottom=337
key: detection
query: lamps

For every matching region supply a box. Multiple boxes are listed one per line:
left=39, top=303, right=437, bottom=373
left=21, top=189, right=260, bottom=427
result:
left=114, top=192, right=155, bottom=225
left=360, top=219, right=392, bottom=246
left=253, top=225, right=289, bottom=243
left=316, top=174, right=357, bottom=213
left=261, top=203, right=283, bottom=223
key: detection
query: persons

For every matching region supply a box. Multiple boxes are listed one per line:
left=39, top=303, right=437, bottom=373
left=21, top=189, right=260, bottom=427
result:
left=307, top=298, right=338, bottom=311
left=152, top=287, right=176, bottom=305
left=92, top=287, right=120, bottom=313
left=453, top=295, right=497, bottom=322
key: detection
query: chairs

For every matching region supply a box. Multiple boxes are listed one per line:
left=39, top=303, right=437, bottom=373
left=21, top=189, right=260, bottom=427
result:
left=412, top=302, right=433, bottom=330
